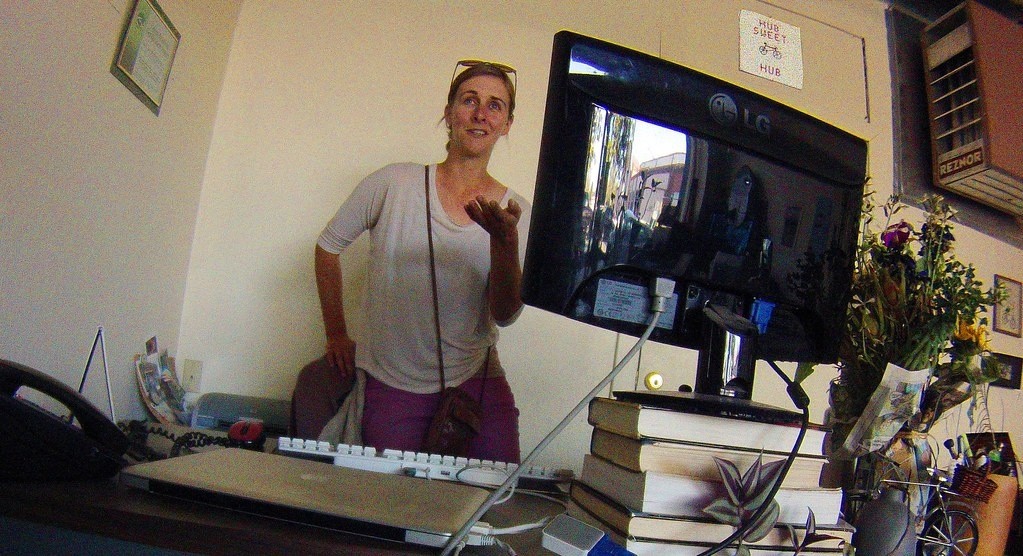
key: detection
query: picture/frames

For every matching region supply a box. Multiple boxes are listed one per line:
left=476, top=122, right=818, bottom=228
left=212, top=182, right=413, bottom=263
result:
left=992, top=273, right=1023, bottom=339
left=989, top=353, right=1023, bottom=390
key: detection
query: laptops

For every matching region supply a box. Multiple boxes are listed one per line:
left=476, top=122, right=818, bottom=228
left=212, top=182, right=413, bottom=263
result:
left=118, top=447, right=491, bottom=548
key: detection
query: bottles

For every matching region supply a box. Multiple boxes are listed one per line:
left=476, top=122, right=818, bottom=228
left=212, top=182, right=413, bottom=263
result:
left=854, top=487, right=918, bottom=556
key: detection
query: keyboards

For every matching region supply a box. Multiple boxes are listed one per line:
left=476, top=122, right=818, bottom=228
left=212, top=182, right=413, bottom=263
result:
left=276, top=437, right=577, bottom=492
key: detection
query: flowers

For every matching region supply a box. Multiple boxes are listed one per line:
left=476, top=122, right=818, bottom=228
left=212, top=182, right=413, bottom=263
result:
left=845, top=174, right=999, bottom=429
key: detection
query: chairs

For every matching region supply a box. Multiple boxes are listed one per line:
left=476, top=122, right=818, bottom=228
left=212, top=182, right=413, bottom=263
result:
left=286, top=352, right=354, bottom=464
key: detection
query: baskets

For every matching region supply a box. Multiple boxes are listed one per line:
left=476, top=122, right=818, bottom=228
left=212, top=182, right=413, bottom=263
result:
left=949, top=452, right=997, bottom=503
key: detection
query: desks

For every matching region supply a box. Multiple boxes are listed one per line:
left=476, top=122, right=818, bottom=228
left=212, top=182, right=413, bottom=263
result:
left=0, top=451, right=568, bottom=556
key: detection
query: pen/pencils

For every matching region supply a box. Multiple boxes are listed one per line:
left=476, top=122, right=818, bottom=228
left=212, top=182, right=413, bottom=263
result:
left=943, top=431, right=1018, bottom=477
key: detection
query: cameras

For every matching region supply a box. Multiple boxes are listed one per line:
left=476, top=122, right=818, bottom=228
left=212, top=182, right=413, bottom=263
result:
left=519, top=30, right=868, bottom=424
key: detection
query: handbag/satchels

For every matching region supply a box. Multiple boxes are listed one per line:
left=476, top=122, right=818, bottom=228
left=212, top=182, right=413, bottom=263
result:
left=420, top=386, right=482, bottom=458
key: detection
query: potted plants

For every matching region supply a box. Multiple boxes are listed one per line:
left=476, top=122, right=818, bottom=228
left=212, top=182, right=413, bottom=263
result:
left=950, top=359, right=1018, bottom=556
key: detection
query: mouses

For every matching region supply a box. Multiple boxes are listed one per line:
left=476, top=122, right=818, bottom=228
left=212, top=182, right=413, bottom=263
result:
left=228, top=418, right=267, bottom=451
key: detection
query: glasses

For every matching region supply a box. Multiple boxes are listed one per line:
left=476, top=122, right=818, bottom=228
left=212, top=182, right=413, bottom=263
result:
left=450, top=60, right=516, bottom=96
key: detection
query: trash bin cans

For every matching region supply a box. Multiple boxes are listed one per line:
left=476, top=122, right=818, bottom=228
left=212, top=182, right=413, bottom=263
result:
left=191, top=395, right=294, bottom=436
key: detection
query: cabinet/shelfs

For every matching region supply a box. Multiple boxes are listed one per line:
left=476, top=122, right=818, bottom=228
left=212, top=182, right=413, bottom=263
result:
left=920, top=0, right=1023, bottom=220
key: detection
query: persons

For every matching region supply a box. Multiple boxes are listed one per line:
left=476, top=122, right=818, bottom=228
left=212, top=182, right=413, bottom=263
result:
left=315, top=63, right=532, bottom=468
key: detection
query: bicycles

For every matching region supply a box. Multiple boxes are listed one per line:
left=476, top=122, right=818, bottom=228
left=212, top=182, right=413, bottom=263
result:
left=842, top=440, right=999, bottom=556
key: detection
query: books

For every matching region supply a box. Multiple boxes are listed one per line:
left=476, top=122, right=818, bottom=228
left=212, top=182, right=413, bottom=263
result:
left=567, top=397, right=857, bottom=556
